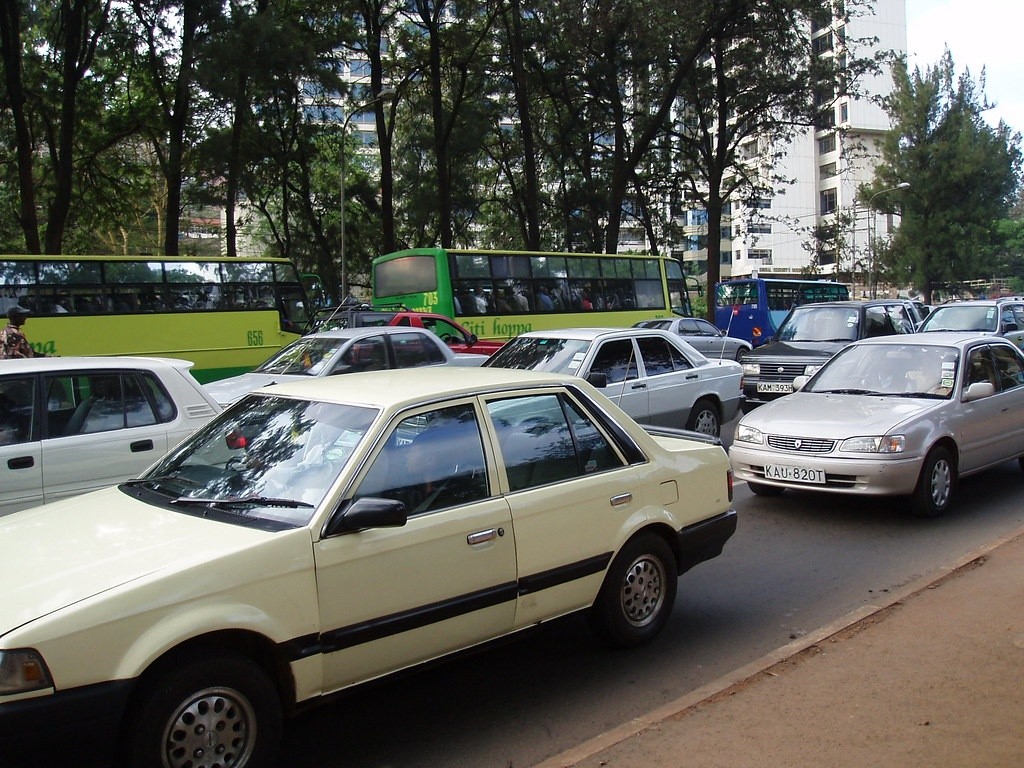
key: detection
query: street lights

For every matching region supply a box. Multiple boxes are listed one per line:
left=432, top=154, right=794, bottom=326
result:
left=867, top=182, right=911, bottom=300
left=339, top=86, right=396, bottom=318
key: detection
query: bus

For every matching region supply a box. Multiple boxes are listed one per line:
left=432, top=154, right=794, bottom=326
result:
left=369, top=247, right=706, bottom=349
left=1, top=255, right=325, bottom=385
left=712, top=278, right=851, bottom=348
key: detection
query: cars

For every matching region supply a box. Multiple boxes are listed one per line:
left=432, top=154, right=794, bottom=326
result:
left=631, top=317, right=754, bottom=373
left=0, top=356, right=249, bottom=516
left=0, top=366, right=738, bottom=768
left=728, top=331, right=1024, bottom=518
left=199, top=325, right=493, bottom=448
left=405, top=328, right=744, bottom=462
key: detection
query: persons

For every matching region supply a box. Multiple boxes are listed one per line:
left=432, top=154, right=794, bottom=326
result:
left=0, top=304, right=57, bottom=360
left=861, top=347, right=1021, bottom=403
left=394, top=427, right=462, bottom=515
left=454, top=281, right=629, bottom=315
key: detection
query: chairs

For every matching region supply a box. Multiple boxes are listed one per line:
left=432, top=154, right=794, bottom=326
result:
left=505, top=295, right=523, bottom=313
left=533, top=296, right=544, bottom=312
left=502, top=432, right=539, bottom=492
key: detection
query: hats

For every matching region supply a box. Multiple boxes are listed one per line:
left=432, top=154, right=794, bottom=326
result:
left=979, top=294, right=987, bottom=300
left=6, top=304, right=30, bottom=317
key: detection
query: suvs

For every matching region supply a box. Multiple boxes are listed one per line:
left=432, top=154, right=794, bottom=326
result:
left=300, top=304, right=509, bottom=377
left=736, top=299, right=928, bottom=414
left=907, top=298, right=1024, bottom=373
